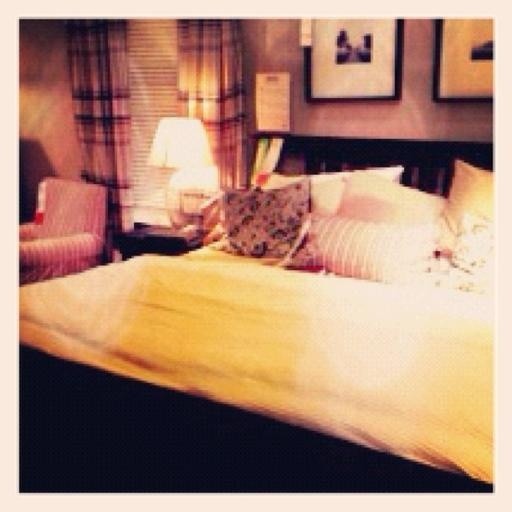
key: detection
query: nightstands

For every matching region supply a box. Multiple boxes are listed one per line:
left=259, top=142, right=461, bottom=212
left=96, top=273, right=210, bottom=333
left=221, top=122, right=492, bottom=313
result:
left=117, top=226, right=205, bottom=259
left=147, top=117, right=206, bottom=231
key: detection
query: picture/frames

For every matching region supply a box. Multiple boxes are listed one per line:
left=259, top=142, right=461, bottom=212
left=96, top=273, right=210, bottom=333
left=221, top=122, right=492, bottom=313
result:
left=308, top=19, right=403, bottom=104
left=432, top=18, right=492, bottom=102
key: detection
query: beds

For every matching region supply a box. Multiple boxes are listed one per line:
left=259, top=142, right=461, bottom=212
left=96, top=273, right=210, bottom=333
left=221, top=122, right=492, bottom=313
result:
left=18, top=136, right=493, bottom=490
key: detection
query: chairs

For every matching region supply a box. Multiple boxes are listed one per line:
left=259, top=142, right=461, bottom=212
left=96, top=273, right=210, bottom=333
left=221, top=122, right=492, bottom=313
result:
left=20, top=177, right=108, bottom=285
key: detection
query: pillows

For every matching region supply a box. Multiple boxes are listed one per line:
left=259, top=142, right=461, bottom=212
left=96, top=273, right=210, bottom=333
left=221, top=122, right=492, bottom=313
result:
left=220, top=156, right=492, bottom=281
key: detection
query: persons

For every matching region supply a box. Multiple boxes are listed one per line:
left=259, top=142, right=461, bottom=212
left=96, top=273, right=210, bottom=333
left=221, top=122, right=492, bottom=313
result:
left=337, top=30, right=372, bottom=63
